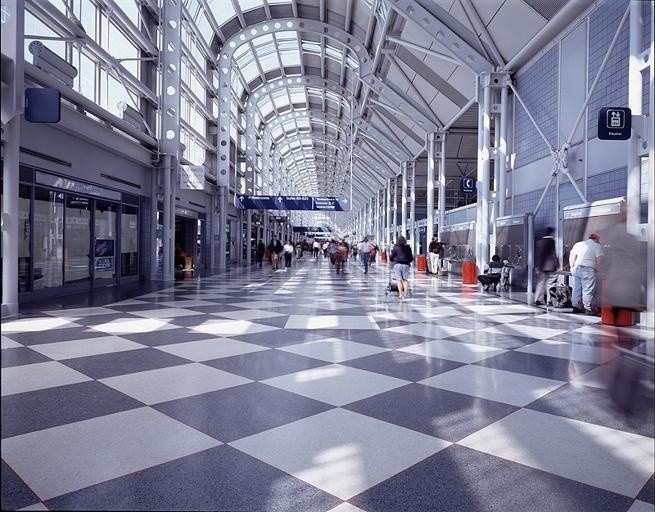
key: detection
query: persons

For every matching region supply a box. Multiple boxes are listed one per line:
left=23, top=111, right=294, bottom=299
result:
left=567, top=233, right=605, bottom=316
left=477, top=254, right=503, bottom=290
left=532, top=227, right=559, bottom=306
left=256, top=234, right=445, bottom=299
left=595, top=196, right=650, bottom=377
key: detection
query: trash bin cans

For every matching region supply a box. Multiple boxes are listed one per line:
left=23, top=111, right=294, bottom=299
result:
left=601, top=281, right=634, bottom=326
left=462, top=255, right=476, bottom=284
left=183, top=256, right=194, bottom=271
left=381, top=251, right=387, bottom=262
left=417, top=254, right=427, bottom=271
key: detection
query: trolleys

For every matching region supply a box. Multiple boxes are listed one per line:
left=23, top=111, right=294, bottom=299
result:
left=330, top=248, right=346, bottom=272
left=385, top=264, right=412, bottom=296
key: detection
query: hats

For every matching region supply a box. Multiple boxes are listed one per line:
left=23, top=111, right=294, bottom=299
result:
left=590, top=232, right=600, bottom=243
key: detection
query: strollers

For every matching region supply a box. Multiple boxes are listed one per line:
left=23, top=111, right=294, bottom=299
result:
left=484, top=264, right=514, bottom=293
